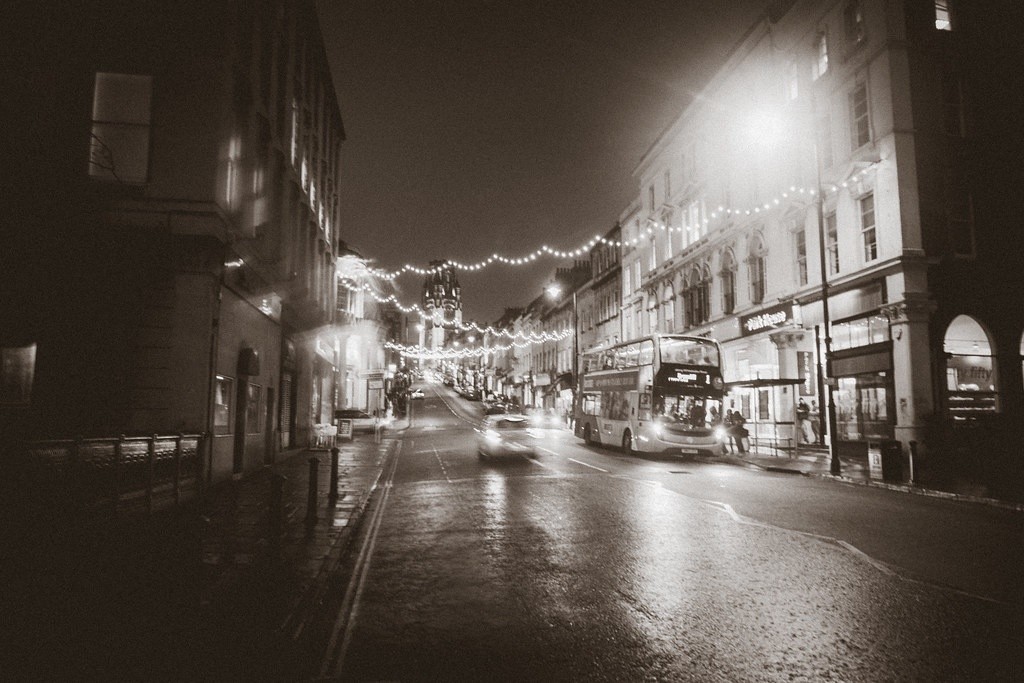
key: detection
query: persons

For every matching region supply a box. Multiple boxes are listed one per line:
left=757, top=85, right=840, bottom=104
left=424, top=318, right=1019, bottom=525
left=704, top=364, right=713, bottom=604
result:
left=583, top=349, right=830, bottom=459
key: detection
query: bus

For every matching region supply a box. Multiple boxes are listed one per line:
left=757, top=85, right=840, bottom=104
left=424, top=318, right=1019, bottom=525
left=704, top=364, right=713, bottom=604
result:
left=569, top=334, right=727, bottom=459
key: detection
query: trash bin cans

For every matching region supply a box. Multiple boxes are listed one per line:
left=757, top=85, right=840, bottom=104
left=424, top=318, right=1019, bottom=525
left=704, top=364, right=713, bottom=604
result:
left=868, top=438, right=903, bottom=482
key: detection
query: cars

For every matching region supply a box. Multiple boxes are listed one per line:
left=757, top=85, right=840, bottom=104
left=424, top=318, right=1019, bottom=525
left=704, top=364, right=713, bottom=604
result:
left=409, top=387, right=425, bottom=399
left=410, top=366, right=453, bottom=386
left=475, top=413, right=533, bottom=461
